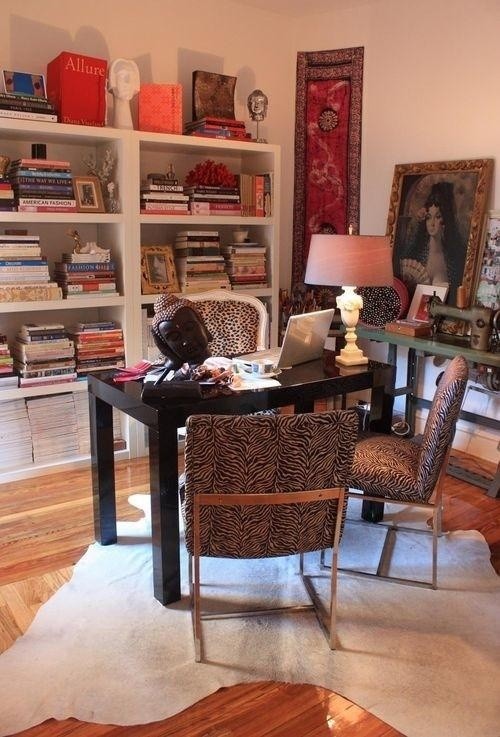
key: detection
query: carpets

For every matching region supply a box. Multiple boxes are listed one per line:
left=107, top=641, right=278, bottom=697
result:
left=0, top=471, right=500, bottom=736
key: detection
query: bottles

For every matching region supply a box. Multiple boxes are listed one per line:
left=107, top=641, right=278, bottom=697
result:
left=457, top=286, right=469, bottom=309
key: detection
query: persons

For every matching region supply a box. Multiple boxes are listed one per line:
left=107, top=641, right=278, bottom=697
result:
left=152, top=293, right=212, bottom=363
left=412, top=183, right=462, bottom=306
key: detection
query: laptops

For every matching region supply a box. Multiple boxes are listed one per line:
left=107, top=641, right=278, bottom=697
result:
left=234, top=308, right=335, bottom=369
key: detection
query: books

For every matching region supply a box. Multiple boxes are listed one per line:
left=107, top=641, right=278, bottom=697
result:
left=1, top=392, right=122, bottom=474
left=140, top=159, right=274, bottom=296
left=0, top=92, right=59, bottom=123
left=182, top=117, right=254, bottom=142
left=0, top=159, right=124, bottom=391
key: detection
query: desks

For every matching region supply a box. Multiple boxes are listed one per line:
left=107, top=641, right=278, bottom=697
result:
left=88, top=350, right=397, bottom=606
left=340, top=324, right=500, bottom=498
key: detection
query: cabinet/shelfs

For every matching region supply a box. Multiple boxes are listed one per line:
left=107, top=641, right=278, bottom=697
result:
left=0, top=117, right=138, bottom=485
left=133, top=131, right=282, bottom=459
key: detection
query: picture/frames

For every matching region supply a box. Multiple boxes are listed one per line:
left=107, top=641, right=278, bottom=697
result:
left=385, top=158, right=500, bottom=341
left=140, top=246, right=181, bottom=294
left=72, top=177, right=105, bottom=213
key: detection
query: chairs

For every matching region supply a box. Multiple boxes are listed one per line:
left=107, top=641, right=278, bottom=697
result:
left=179, top=290, right=270, bottom=356
left=320, top=356, right=468, bottom=590
left=181, top=410, right=360, bottom=663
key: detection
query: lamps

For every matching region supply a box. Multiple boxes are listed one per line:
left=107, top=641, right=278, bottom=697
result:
left=305, top=225, right=394, bottom=367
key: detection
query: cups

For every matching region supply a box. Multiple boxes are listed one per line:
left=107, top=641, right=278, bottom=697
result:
left=251, top=358, right=274, bottom=375
left=32, top=144, right=46, bottom=160
left=233, top=232, right=248, bottom=242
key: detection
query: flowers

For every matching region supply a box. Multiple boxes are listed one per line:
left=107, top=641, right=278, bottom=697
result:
left=185, top=159, right=237, bottom=187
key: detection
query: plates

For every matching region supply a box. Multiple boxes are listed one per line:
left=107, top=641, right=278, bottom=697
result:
left=229, top=243, right=259, bottom=246
left=250, top=369, right=283, bottom=379
